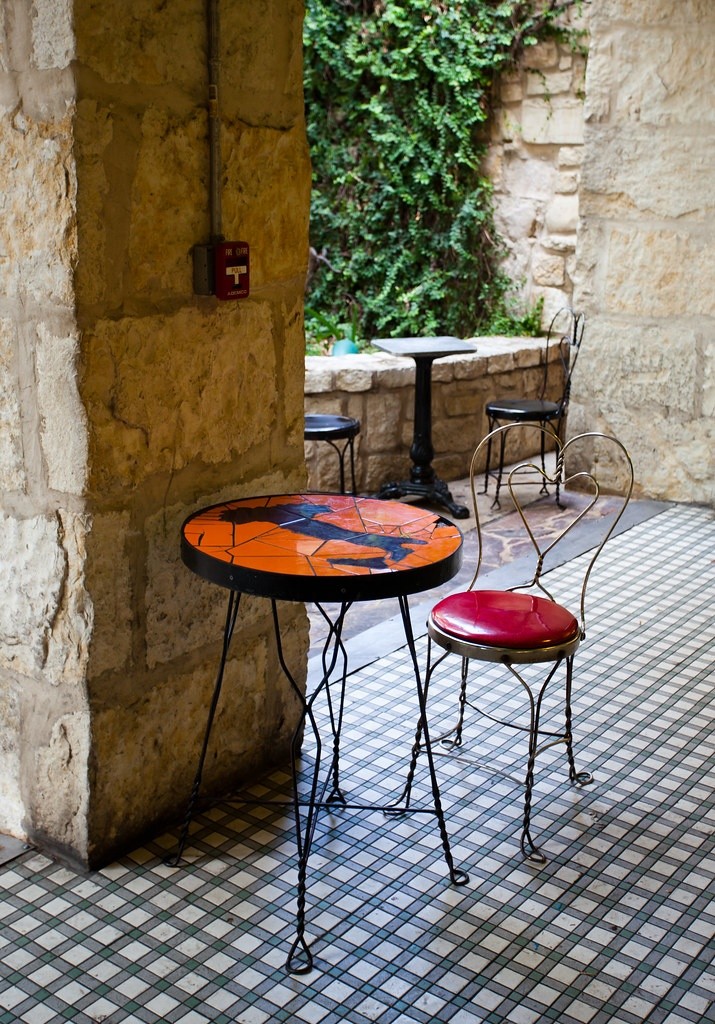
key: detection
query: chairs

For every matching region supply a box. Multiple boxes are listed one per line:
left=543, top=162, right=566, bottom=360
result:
left=478, top=307, right=586, bottom=511
left=383, top=422, right=635, bottom=863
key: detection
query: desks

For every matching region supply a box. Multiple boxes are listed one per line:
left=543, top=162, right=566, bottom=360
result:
left=369, top=336, right=477, bottom=520
left=161, top=493, right=470, bottom=976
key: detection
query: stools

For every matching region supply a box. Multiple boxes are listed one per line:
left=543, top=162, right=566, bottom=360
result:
left=304, top=414, right=361, bottom=496
